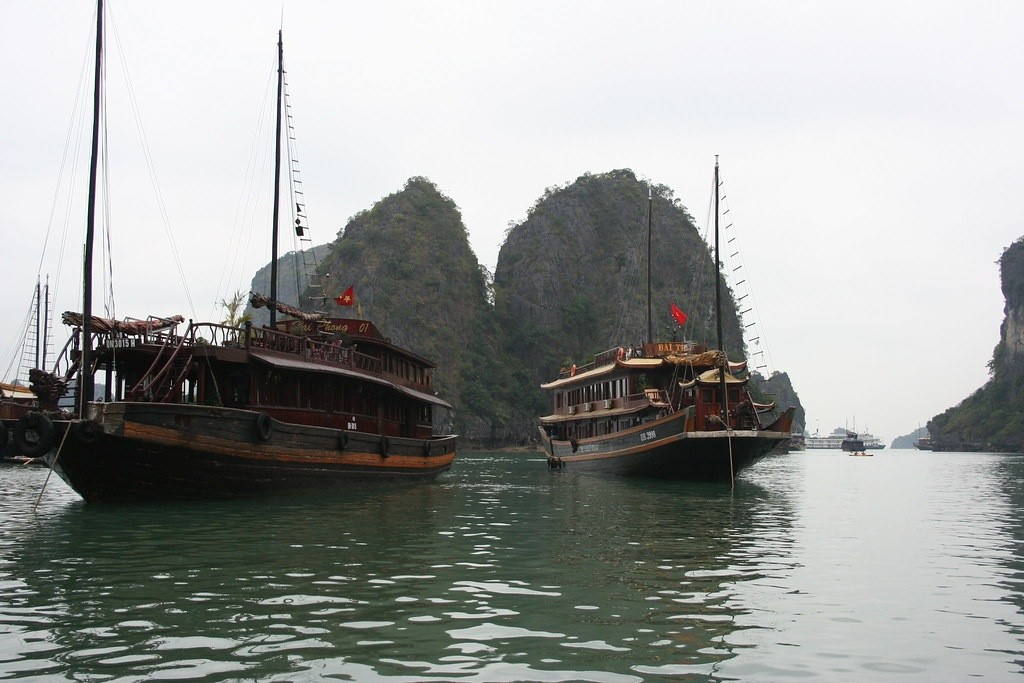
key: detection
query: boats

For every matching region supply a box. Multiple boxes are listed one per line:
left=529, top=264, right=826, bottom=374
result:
left=804, top=414, right=886, bottom=450
left=848, top=449, right=874, bottom=457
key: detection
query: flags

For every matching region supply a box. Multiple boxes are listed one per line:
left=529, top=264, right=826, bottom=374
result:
left=671, top=303, right=687, bottom=326
left=335, top=287, right=354, bottom=307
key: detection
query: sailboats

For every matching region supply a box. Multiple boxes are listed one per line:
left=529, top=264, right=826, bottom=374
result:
left=0, top=3, right=459, bottom=515
left=537, top=153, right=798, bottom=486
left=913, top=423, right=935, bottom=451
left=841, top=413, right=867, bottom=453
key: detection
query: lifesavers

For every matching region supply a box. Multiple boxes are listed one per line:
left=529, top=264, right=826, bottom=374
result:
left=340, top=432, right=349, bottom=449
left=569, top=435, right=577, bottom=450
left=570, top=364, right=576, bottom=376
left=617, top=347, right=624, bottom=360
left=257, top=411, right=272, bottom=440
left=380, top=437, right=390, bottom=458
left=13, top=412, right=56, bottom=458
left=76, top=420, right=100, bottom=444
left=425, top=441, right=431, bottom=455
left=0, top=421, right=8, bottom=448
